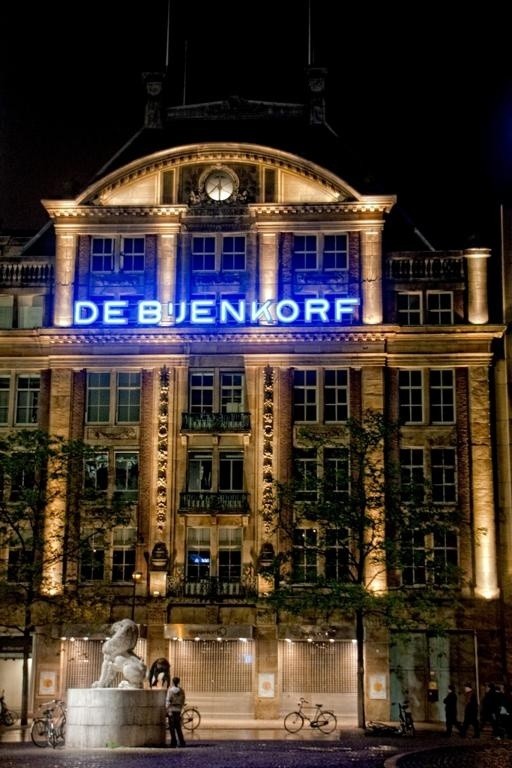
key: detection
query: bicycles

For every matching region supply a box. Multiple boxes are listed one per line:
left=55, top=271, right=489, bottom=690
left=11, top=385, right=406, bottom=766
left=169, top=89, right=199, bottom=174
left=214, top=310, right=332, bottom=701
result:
left=30, top=700, right=66, bottom=748
left=166, top=700, right=200, bottom=731
left=284, top=697, right=336, bottom=733
left=392, top=701, right=414, bottom=735
left=0, top=691, right=18, bottom=726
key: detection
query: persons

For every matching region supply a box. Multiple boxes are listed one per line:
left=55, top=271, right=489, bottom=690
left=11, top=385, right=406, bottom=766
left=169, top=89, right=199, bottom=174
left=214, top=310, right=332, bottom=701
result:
left=443, top=683, right=512, bottom=740
left=166, top=677, right=186, bottom=748
left=400, top=690, right=414, bottom=728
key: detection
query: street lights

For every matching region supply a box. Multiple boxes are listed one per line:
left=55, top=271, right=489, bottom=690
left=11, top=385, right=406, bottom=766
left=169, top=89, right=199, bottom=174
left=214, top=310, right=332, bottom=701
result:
left=131, top=571, right=142, bottom=623
